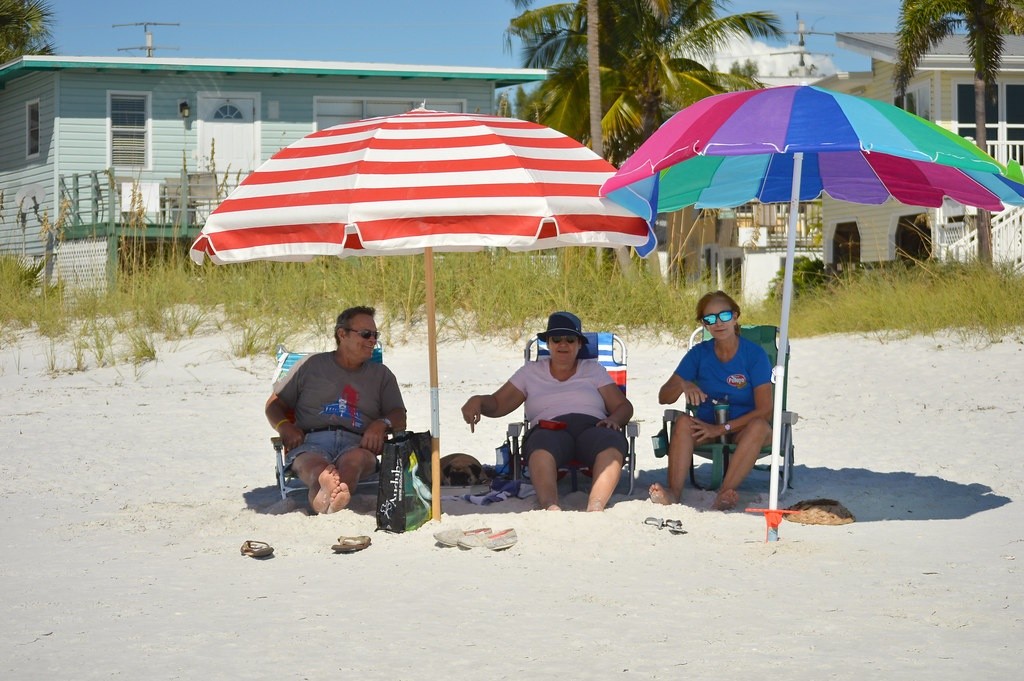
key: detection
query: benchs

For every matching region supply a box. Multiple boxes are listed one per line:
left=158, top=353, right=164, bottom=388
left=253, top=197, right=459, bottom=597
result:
left=664, top=324, right=798, bottom=496
left=507, top=333, right=638, bottom=496
left=269, top=347, right=393, bottom=500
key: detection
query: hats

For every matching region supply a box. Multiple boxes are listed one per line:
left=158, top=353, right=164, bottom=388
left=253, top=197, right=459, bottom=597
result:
left=537, top=311, right=588, bottom=344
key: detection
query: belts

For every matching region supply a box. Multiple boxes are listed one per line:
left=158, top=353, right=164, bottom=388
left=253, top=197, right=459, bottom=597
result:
left=304, top=426, right=362, bottom=435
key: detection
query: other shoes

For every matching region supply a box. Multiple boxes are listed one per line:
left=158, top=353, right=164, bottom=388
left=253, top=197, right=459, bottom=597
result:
left=432, top=528, right=492, bottom=546
left=458, top=528, right=517, bottom=549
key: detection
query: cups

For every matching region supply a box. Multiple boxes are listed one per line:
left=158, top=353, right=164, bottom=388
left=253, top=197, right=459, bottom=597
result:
left=714, top=405, right=730, bottom=445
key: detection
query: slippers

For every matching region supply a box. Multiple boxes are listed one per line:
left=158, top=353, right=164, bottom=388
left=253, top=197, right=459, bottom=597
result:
left=332, top=535, right=372, bottom=552
left=241, top=541, right=272, bottom=556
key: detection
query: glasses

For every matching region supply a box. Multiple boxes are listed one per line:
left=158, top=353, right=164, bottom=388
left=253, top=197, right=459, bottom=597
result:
left=702, top=310, right=733, bottom=325
left=552, top=336, right=578, bottom=344
left=344, top=327, right=380, bottom=339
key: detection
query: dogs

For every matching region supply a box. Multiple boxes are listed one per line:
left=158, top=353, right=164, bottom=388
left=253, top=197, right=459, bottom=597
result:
left=439, top=452, right=485, bottom=486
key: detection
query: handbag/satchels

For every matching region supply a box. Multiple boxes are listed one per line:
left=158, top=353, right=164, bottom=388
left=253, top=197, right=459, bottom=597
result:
left=376, top=430, right=432, bottom=533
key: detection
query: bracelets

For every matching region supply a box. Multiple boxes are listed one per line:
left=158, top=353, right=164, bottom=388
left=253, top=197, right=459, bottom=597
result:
left=275, top=418, right=290, bottom=429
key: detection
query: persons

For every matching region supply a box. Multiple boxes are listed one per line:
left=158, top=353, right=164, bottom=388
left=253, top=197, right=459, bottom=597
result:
left=462, top=312, right=634, bottom=511
left=265, top=306, right=406, bottom=514
left=649, top=290, right=774, bottom=509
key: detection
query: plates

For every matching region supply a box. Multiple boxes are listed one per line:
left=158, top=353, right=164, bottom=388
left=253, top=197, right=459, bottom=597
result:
left=538, top=419, right=567, bottom=430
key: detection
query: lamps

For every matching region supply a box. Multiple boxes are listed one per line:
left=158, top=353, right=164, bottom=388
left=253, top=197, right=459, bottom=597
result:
left=179, top=100, right=189, bottom=118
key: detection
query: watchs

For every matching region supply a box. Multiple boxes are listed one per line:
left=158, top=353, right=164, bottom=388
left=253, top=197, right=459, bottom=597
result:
left=724, top=423, right=731, bottom=433
left=377, top=417, right=393, bottom=433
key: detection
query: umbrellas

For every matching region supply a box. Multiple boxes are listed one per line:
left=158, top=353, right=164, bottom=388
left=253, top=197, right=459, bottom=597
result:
left=189, top=109, right=650, bottom=522
left=601, top=86, right=1024, bottom=509
